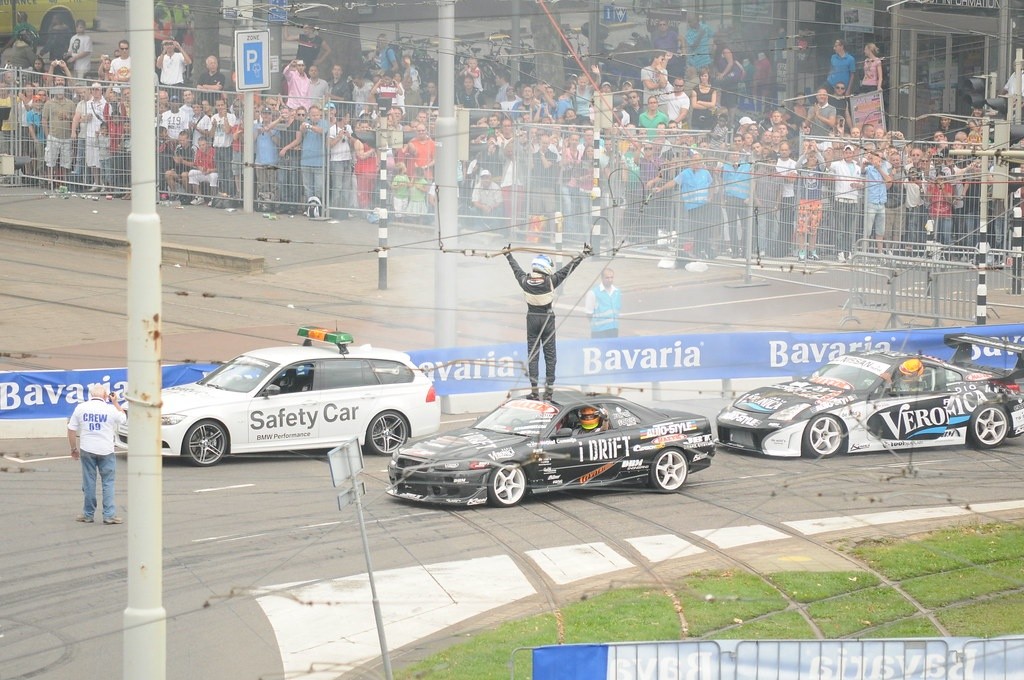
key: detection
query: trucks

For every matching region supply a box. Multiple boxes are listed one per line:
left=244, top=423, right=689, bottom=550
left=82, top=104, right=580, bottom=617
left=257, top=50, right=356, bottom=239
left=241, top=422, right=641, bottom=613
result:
left=0, top=1, right=100, bottom=46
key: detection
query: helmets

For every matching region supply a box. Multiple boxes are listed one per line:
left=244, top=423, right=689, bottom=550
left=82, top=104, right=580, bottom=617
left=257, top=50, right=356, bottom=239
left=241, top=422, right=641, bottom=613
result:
left=898, top=359, right=925, bottom=383
left=579, top=407, right=600, bottom=430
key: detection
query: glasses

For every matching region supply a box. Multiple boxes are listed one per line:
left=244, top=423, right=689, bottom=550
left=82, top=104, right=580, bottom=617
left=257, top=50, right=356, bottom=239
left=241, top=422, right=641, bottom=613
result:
left=120, top=47, right=129, bottom=50
left=674, top=83, right=684, bottom=88
left=835, top=87, right=845, bottom=90
left=270, top=104, right=277, bottom=106
left=297, top=114, right=305, bottom=116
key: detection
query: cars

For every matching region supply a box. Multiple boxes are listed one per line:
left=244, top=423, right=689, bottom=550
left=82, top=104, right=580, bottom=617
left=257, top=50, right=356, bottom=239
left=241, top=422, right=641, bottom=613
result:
left=385, top=390, right=715, bottom=509
left=714, top=332, right=1024, bottom=460
left=114, top=327, right=441, bottom=468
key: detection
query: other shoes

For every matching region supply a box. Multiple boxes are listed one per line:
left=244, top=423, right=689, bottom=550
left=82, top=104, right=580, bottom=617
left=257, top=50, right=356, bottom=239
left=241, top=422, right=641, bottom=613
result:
left=798, top=249, right=819, bottom=260
left=88, top=186, right=105, bottom=192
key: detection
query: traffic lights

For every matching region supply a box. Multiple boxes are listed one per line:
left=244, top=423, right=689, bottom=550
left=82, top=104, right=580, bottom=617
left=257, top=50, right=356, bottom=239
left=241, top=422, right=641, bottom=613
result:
left=351, top=131, right=381, bottom=152
left=993, top=121, right=1024, bottom=199
left=458, top=110, right=489, bottom=162
left=983, top=94, right=1013, bottom=143
left=389, top=131, right=415, bottom=149
left=964, top=76, right=987, bottom=109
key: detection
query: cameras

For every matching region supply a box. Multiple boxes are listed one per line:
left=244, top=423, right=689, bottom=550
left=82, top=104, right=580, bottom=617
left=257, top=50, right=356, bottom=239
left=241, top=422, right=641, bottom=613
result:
left=594, top=408, right=607, bottom=420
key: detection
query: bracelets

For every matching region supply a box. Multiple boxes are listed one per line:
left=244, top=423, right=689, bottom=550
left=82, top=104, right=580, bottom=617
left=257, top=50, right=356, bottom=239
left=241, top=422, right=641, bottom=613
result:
left=424, top=165, right=428, bottom=170
left=658, top=70, right=663, bottom=74
left=66, top=114, right=70, bottom=121
left=305, top=124, right=312, bottom=131
left=70, top=448, right=77, bottom=451
left=161, top=51, right=165, bottom=56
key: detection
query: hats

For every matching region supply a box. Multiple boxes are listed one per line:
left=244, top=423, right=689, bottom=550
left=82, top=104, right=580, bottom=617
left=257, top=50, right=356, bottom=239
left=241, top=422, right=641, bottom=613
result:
left=531, top=254, right=555, bottom=276
left=739, top=116, right=756, bottom=126
left=91, top=82, right=101, bottom=89
left=601, top=82, right=611, bottom=87
left=480, top=170, right=491, bottom=176
left=169, top=95, right=181, bottom=101
left=262, top=107, right=271, bottom=113
left=843, top=145, right=855, bottom=152
left=622, top=80, right=632, bottom=86
left=326, top=103, right=335, bottom=109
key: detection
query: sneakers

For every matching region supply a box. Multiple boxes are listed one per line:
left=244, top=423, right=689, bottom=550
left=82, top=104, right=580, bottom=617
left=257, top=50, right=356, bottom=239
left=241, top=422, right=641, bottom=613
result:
left=543, top=392, right=553, bottom=400
left=190, top=197, right=205, bottom=205
left=103, top=514, right=123, bottom=523
left=207, top=198, right=213, bottom=206
left=836, top=251, right=845, bottom=263
left=76, top=514, right=94, bottom=522
left=526, top=393, right=539, bottom=400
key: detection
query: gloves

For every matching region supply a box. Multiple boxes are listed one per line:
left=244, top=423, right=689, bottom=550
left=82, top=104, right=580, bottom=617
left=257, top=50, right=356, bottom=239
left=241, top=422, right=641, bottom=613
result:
left=502, top=244, right=511, bottom=256
left=583, top=242, right=594, bottom=257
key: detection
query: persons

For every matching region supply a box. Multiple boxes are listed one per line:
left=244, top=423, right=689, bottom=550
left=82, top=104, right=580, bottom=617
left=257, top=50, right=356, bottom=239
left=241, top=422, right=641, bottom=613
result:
left=0, top=4, right=1024, bottom=263
left=585, top=267, right=622, bottom=339
left=68, top=385, right=127, bottom=524
left=502, top=242, right=593, bottom=400
left=881, top=359, right=924, bottom=396
left=571, top=408, right=609, bottom=436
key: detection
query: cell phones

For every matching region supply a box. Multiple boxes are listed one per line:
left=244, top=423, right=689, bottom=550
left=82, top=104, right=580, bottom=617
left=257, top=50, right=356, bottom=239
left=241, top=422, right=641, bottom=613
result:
left=103, top=55, right=109, bottom=59
left=109, top=392, right=114, bottom=402
left=297, top=59, right=303, bottom=64
left=840, top=119, right=844, bottom=127
left=165, top=42, right=174, bottom=47
left=55, top=61, right=61, bottom=65
